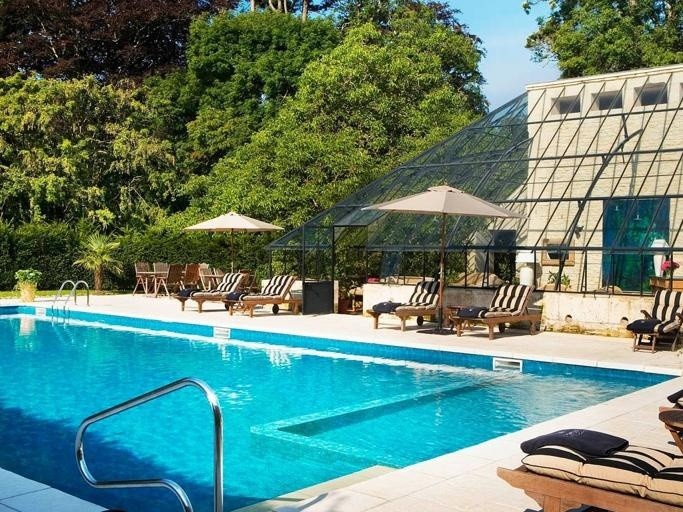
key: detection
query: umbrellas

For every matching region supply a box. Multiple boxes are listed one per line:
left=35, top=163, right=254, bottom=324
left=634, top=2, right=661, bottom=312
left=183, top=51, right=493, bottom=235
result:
left=359, top=183, right=530, bottom=330
left=182, top=211, right=284, bottom=272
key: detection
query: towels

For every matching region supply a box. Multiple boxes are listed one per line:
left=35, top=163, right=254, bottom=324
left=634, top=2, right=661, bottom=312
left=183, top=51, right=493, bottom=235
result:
left=668, top=390, right=683, bottom=403
left=627, top=319, right=661, bottom=333
left=521, top=429, right=629, bottom=458
left=459, top=306, right=487, bottom=318
left=373, top=302, right=401, bottom=313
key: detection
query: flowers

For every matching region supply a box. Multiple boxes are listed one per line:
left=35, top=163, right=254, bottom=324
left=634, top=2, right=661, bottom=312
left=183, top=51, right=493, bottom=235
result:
left=661, top=261, right=680, bottom=278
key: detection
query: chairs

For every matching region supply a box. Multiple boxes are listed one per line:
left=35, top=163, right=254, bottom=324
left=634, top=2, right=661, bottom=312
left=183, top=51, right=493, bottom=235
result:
left=135, top=260, right=303, bottom=317
left=660, top=389, right=683, bottom=437
left=366, top=281, right=440, bottom=332
left=455, top=285, right=542, bottom=340
left=627, top=289, right=683, bottom=354
left=497, top=413, right=683, bottom=512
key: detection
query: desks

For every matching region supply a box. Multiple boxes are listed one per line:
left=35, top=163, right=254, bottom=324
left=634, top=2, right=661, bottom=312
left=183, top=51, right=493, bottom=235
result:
left=447, top=305, right=473, bottom=331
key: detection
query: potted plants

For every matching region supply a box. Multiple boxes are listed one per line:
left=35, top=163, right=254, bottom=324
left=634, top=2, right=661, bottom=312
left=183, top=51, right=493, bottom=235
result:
left=338, top=279, right=357, bottom=314
left=14, top=268, right=42, bottom=301
